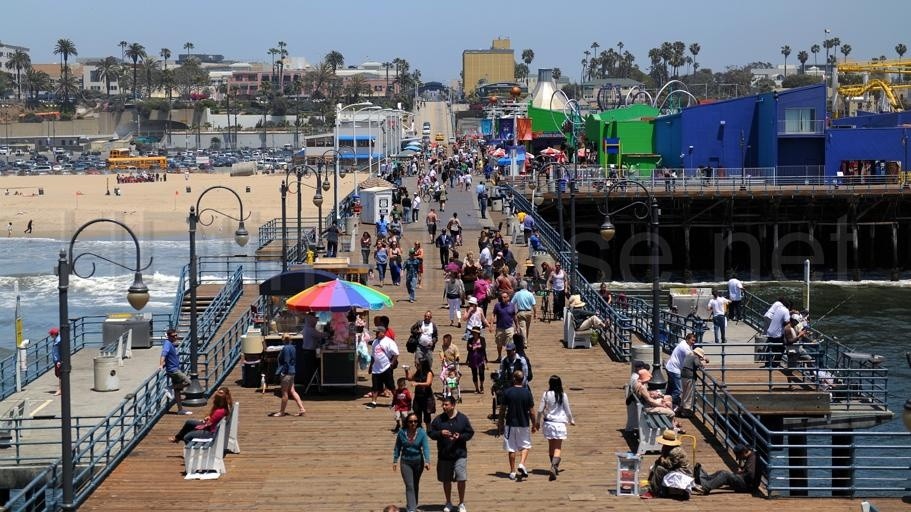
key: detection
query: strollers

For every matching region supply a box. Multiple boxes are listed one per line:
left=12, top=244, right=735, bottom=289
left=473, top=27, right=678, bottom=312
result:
left=442, top=356, right=463, bottom=403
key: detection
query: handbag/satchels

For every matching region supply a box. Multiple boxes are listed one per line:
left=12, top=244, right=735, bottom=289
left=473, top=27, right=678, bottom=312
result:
left=406, top=334, right=419, bottom=353
left=389, top=356, right=399, bottom=370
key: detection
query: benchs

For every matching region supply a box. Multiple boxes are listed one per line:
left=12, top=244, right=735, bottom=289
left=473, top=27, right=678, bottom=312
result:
left=598, top=185, right=627, bottom=192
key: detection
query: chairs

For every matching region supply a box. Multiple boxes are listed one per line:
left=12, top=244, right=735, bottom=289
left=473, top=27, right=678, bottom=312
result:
left=341, top=230, right=355, bottom=252
left=185, top=401, right=241, bottom=480
left=0, top=399, right=25, bottom=449
left=624, top=383, right=681, bottom=457
left=97, top=329, right=133, bottom=367
left=512, top=223, right=525, bottom=244
left=563, top=308, right=592, bottom=349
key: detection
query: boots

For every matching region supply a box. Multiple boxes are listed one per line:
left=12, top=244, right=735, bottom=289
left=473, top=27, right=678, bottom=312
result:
left=549, top=454, right=561, bottom=481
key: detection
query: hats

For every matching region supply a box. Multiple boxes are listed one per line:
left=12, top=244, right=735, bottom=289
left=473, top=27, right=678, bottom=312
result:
left=654, top=430, right=681, bottom=446
left=734, top=443, right=749, bottom=460
left=468, top=296, right=478, bottom=306
left=791, top=314, right=801, bottom=324
left=471, top=327, right=480, bottom=333
left=568, top=293, right=580, bottom=303
left=637, top=368, right=652, bottom=382
left=522, top=259, right=535, bottom=267
left=570, top=299, right=586, bottom=308
left=373, top=326, right=386, bottom=332
left=505, top=343, right=516, bottom=350
left=692, top=347, right=705, bottom=358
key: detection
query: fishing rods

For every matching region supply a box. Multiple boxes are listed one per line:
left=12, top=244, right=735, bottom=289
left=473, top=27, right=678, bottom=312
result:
left=831, top=332, right=854, bottom=376
left=803, top=293, right=856, bottom=330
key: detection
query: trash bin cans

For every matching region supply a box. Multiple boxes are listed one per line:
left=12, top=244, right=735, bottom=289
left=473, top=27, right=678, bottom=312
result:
left=506, top=217, right=519, bottom=236
left=246, top=186, right=250, bottom=192
left=186, top=186, right=191, bottom=192
left=93, top=355, right=120, bottom=391
left=114, top=188, right=120, bottom=196
left=345, top=217, right=358, bottom=236
left=491, top=196, right=503, bottom=212
left=557, top=179, right=567, bottom=192
left=754, top=335, right=770, bottom=363
left=39, top=188, right=43, bottom=195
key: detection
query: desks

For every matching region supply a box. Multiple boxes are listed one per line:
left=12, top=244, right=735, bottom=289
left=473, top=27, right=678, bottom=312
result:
left=264, top=332, right=359, bottom=389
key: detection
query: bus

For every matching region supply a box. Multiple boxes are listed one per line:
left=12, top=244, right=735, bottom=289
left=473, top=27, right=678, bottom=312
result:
left=19, top=111, right=61, bottom=123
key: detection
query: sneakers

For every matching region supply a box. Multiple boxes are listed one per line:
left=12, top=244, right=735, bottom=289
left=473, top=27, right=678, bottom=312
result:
left=444, top=502, right=453, bottom=512
left=517, top=464, right=529, bottom=477
left=366, top=402, right=377, bottom=408
left=293, top=409, right=307, bottom=416
left=457, top=504, right=466, bottom=512
left=162, top=386, right=173, bottom=399
left=509, top=472, right=517, bottom=479
left=178, top=409, right=193, bottom=415
left=272, top=411, right=286, bottom=417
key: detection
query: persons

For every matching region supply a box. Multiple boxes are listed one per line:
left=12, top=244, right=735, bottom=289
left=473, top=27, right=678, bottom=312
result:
left=670, top=291, right=730, bottom=342
left=728, top=274, right=744, bottom=320
left=442, top=209, right=567, bottom=349
left=393, top=414, right=430, bottom=512
left=648, top=430, right=760, bottom=500
left=168, top=387, right=231, bottom=444
left=858, top=99, right=900, bottom=112
left=1, top=101, right=724, bottom=192
left=498, top=189, right=514, bottom=209
left=763, top=297, right=811, bottom=367
left=568, top=283, right=611, bottom=330
left=48, top=329, right=61, bottom=395
left=498, top=370, right=537, bottom=480
left=428, top=396, right=475, bottom=512
left=631, top=361, right=686, bottom=433
left=841, top=160, right=898, bottom=185
left=666, top=334, right=709, bottom=418
left=272, top=333, right=305, bottom=416
left=158, top=329, right=192, bottom=414
left=267, top=311, right=364, bottom=395
left=364, top=292, right=533, bottom=433
left=477, top=181, right=490, bottom=219
left=536, top=376, right=576, bottom=479
left=321, top=185, right=462, bottom=302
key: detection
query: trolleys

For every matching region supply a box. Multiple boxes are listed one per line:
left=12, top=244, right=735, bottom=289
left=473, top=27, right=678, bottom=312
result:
left=678, top=434, right=701, bottom=483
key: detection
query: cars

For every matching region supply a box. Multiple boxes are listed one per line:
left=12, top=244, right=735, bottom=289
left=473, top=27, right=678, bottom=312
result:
left=436, top=131, right=445, bottom=141
left=0, top=142, right=295, bottom=176
left=448, top=137, right=456, bottom=144
left=422, top=121, right=432, bottom=134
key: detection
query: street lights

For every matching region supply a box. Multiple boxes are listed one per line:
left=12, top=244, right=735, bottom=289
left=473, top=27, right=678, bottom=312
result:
left=489, top=96, right=498, bottom=150
left=353, top=106, right=382, bottom=207
left=51, top=216, right=156, bottom=511
left=511, top=87, right=522, bottom=182
left=396, top=111, right=416, bottom=157
left=278, top=164, right=325, bottom=275
left=529, top=153, right=565, bottom=265
left=597, top=178, right=670, bottom=397
left=385, top=109, right=399, bottom=165
left=333, top=101, right=373, bottom=221
left=179, top=185, right=252, bottom=407
left=368, top=107, right=393, bottom=177
left=332, top=145, right=359, bottom=228
left=533, top=163, right=578, bottom=300
left=315, top=150, right=347, bottom=254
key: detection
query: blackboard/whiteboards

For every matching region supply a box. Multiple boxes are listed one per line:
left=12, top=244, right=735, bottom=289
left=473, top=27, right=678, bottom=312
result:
left=320, top=350, right=357, bottom=386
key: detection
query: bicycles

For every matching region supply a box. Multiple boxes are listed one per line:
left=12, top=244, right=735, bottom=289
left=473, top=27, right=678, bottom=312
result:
left=535, top=288, right=557, bottom=323
left=422, top=186, right=440, bottom=203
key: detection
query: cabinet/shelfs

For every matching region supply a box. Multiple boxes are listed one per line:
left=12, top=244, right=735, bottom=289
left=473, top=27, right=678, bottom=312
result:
left=617, top=456, right=639, bottom=497
